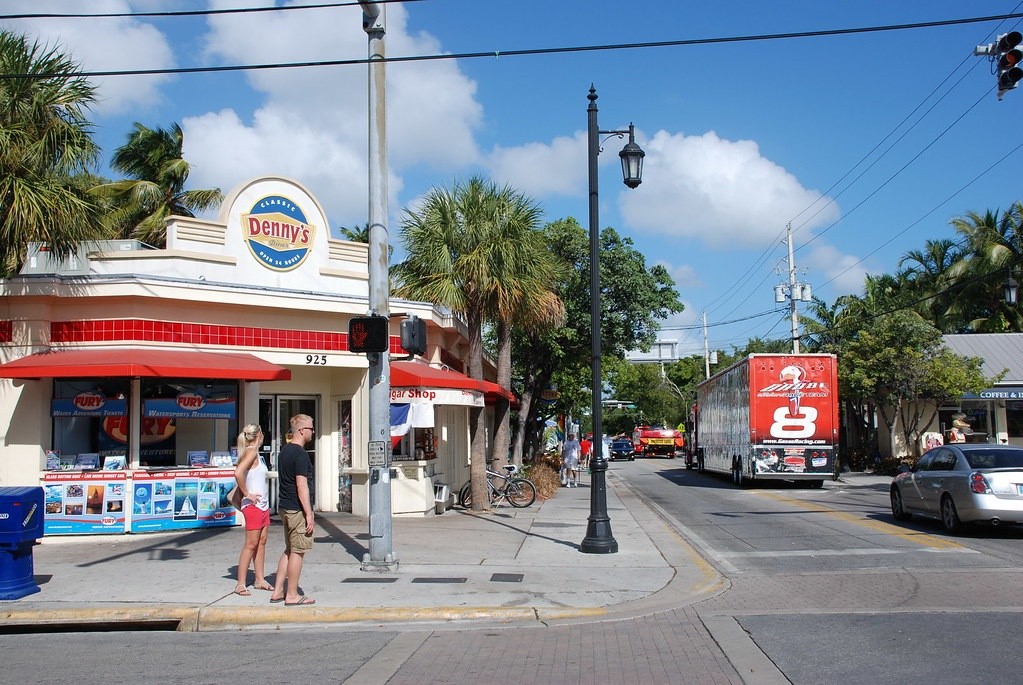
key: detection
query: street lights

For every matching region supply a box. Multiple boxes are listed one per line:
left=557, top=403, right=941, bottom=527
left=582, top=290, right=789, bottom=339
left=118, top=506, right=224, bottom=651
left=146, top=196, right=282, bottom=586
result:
left=577, top=84, right=645, bottom=553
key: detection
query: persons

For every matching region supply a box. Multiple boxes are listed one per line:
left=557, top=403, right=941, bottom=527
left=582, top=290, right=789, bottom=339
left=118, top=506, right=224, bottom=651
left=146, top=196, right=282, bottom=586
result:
left=579, top=436, right=591, bottom=468
left=234, top=423, right=274, bottom=596
left=562, top=433, right=581, bottom=488
left=270, top=414, right=315, bottom=606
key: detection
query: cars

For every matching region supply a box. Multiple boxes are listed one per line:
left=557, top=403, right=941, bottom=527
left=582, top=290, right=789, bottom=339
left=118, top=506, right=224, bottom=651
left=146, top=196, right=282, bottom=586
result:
left=609, top=442, right=634, bottom=461
left=890, top=443, right=1023, bottom=531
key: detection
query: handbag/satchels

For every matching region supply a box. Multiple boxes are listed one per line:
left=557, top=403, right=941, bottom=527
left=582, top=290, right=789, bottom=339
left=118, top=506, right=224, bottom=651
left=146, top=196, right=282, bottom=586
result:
left=559, top=464, right=566, bottom=485
left=227, top=484, right=244, bottom=511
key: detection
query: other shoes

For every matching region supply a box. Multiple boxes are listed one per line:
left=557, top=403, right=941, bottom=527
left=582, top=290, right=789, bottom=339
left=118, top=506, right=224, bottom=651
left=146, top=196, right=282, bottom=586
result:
left=566, top=484, right=570, bottom=488
left=574, top=483, right=577, bottom=488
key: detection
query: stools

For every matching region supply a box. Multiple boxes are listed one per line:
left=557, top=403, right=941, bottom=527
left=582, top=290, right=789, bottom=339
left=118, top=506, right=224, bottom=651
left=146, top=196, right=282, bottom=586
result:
left=434, top=484, right=449, bottom=502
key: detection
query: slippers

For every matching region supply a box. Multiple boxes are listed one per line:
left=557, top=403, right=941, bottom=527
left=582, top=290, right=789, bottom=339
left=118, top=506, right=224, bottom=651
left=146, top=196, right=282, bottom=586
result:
left=270, top=592, right=286, bottom=603
left=285, top=595, right=315, bottom=606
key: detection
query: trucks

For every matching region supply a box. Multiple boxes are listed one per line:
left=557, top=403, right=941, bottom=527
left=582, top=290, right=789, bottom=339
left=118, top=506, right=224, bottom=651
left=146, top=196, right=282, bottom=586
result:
left=634, top=426, right=676, bottom=459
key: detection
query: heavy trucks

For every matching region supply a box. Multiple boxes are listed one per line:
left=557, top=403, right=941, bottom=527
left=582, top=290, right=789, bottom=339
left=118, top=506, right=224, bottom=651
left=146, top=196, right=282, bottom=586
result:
left=683, top=353, right=840, bottom=489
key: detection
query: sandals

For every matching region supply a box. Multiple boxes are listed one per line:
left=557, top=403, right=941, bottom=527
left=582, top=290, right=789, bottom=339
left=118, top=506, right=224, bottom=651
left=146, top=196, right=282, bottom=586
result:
left=235, top=585, right=251, bottom=596
left=254, top=577, right=275, bottom=591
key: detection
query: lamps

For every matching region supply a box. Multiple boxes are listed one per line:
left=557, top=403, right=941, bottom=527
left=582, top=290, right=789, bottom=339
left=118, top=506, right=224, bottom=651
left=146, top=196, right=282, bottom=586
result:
left=998, top=402, right=1005, bottom=407
left=1002, top=439, right=1007, bottom=443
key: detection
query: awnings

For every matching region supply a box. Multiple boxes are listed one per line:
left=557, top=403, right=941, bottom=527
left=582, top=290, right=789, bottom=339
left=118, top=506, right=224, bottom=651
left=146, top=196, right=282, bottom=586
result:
left=0, top=349, right=291, bottom=383
left=389, top=361, right=517, bottom=405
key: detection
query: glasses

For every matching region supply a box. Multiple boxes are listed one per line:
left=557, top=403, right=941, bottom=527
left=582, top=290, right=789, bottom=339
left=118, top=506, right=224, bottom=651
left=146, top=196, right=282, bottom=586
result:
left=259, top=432, right=265, bottom=436
left=299, top=427, right=314, bottom=433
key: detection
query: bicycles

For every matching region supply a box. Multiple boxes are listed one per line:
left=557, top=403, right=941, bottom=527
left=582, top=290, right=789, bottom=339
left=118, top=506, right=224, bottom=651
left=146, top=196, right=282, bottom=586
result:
left=459, top=457, right=536, bottom=509
left=970, top=433, right=1007, bottom=463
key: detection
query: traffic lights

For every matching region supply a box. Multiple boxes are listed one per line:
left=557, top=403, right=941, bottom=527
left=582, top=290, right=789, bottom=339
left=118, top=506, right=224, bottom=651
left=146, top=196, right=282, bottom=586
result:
left=401, top=316, right=426, bottom=356
left=347, top=317, right=388, bottom=353
left=357, top=0, right=386, bottom=32
left=996, top=31, right=1023, bottom=90
